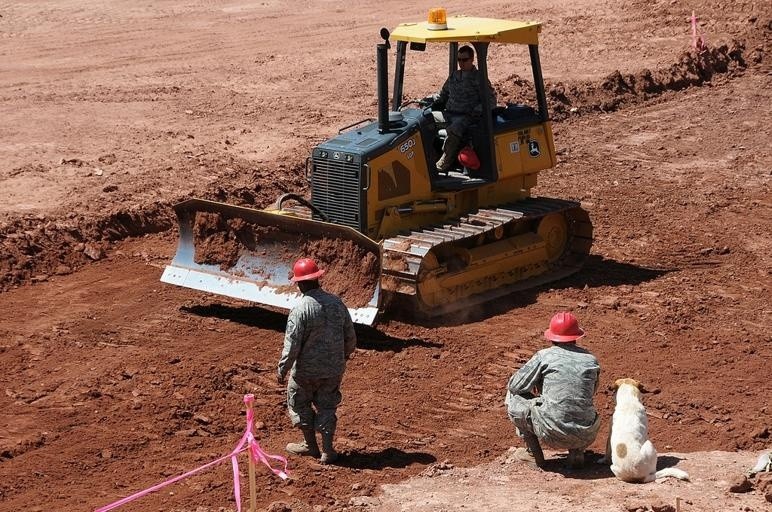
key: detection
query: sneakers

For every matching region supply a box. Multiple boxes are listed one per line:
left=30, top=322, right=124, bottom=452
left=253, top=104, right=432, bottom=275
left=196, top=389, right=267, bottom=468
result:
left=571, top=452, right=584, bottom=469
left=286, top=441, right=320, bottom=456
left=436, top=158, right=455, bottom=170
left=321, top=451, right=337, bottom=463
left=534, top=452, right=544, bottom=465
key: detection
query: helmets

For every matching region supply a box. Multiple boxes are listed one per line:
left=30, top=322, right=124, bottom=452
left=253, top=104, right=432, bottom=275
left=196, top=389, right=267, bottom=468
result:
left=458, top=147, right=481, bottom=170
left=291, top=258, right=325, bottom=282
left=544, top=312, right=586, bottom=343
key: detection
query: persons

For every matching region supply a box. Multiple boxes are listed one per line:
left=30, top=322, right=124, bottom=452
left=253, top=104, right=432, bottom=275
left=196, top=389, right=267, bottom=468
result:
left=503, top=311, right=602, bottom=471
left=420, top=43, right=499, bottom=175
left=276, top=256, right=358, bottom=464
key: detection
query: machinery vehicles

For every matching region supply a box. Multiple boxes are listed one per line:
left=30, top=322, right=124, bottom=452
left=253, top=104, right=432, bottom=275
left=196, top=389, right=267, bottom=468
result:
left=158, top=8, right=592, bottom=330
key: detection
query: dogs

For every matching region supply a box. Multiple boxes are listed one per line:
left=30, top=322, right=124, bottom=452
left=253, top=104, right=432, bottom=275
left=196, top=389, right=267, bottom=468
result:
left=592, top=377, right=694, bottom=486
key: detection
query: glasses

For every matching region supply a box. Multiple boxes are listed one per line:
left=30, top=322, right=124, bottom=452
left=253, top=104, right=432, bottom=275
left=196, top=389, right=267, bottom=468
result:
left=457, top=58, right=471, bottom=62
